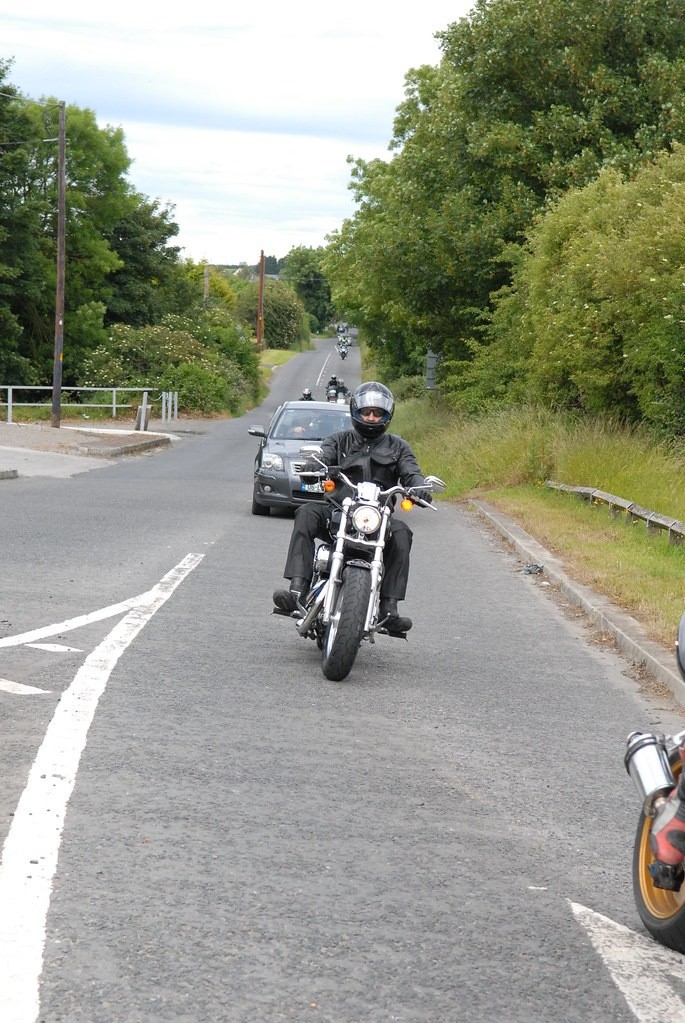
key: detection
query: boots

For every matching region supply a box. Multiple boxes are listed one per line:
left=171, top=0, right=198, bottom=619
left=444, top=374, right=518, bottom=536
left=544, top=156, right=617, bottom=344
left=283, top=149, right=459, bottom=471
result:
left=378, top=597, right=412, bottom=632
left=273, top=576, right=308, bottom=610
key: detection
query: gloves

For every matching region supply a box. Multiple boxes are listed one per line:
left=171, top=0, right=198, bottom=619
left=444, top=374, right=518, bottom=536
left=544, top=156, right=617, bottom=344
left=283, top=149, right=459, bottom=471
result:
left=412, top=488, right=433, bottom=508
left=304, top=461, right=322, bottom=481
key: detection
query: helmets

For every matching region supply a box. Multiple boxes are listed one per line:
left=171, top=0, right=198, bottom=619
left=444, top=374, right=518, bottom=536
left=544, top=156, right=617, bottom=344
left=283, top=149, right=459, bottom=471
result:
left=338, top=378, right=344, bottom=387
left=302, top=389, right=311, bottom=400
left=350, top=382, right=395, bottom=438
left=331, top=375, right=336, bottom=381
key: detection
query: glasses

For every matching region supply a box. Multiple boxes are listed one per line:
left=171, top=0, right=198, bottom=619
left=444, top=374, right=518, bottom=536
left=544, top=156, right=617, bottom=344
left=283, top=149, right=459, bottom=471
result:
left=360, top=408, right=385, bottom=416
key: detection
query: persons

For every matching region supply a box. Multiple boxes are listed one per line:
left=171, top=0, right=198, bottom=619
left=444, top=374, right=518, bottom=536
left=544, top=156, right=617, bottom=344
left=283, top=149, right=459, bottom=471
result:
left=298, top=387, right=315, bottom=402
left=336, top=323, right=349, bottom=332
left=288, top=426, right=306, bottom=433
left=326, top=374, right=340, bottom=399
left=337, top=334, right=349, bottom=357
left=273, top=381, right=433, bottom=633
left=338, top=378, right=348, bottom=395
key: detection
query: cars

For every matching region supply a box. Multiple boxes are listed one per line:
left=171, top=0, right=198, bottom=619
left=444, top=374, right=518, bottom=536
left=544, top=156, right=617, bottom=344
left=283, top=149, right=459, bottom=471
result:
left=247, top=400, right=353, bottom=516
left=335, top=325, right=345, bottom=333
left=337, top=334, right=354, bottom=347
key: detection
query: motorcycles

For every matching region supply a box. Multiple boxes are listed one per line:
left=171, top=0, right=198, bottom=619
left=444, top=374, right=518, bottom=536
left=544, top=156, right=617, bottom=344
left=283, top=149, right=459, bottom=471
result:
left=270, top=447, right=450, bottom=680
left=338, top=345, right=348, bottom=360
left=622, top=612, right=685, bottom=954
left=325, top=385, right=351, bottom=404
